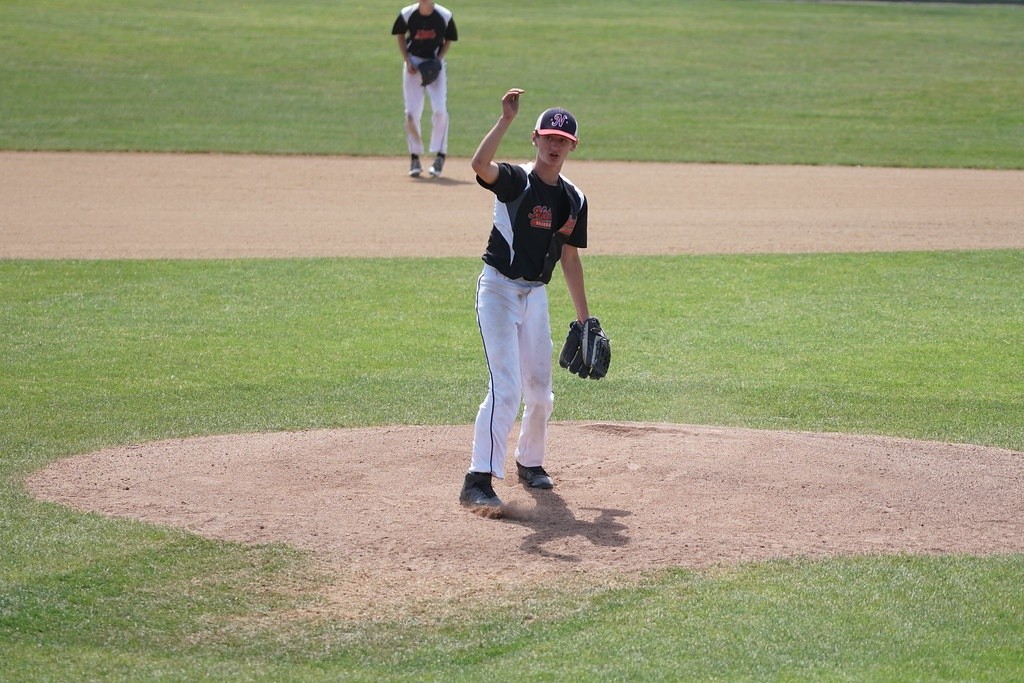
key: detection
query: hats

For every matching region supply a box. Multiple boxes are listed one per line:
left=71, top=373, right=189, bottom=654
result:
left=535, top=107, right=578, bottom=141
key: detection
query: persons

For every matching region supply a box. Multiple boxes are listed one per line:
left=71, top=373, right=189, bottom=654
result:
left=391, top=0, right=458, bottom=176
left=458, top=88, right=611, bottom=515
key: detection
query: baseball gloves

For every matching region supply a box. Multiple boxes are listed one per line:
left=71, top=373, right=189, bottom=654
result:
left=417, top=56, right=443, bottom=87
left=558, top=315, right=612, bottom=382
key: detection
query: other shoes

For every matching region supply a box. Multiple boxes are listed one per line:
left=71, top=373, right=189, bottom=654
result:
left=429, top=155, right=444, bottom=176
left=410, top=159, right=422, bottom=176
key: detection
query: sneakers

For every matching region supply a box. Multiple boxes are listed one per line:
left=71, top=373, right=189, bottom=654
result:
left=516, top=461, right=553, bottom=489
left=459, top=471, right=503, bottom=509
